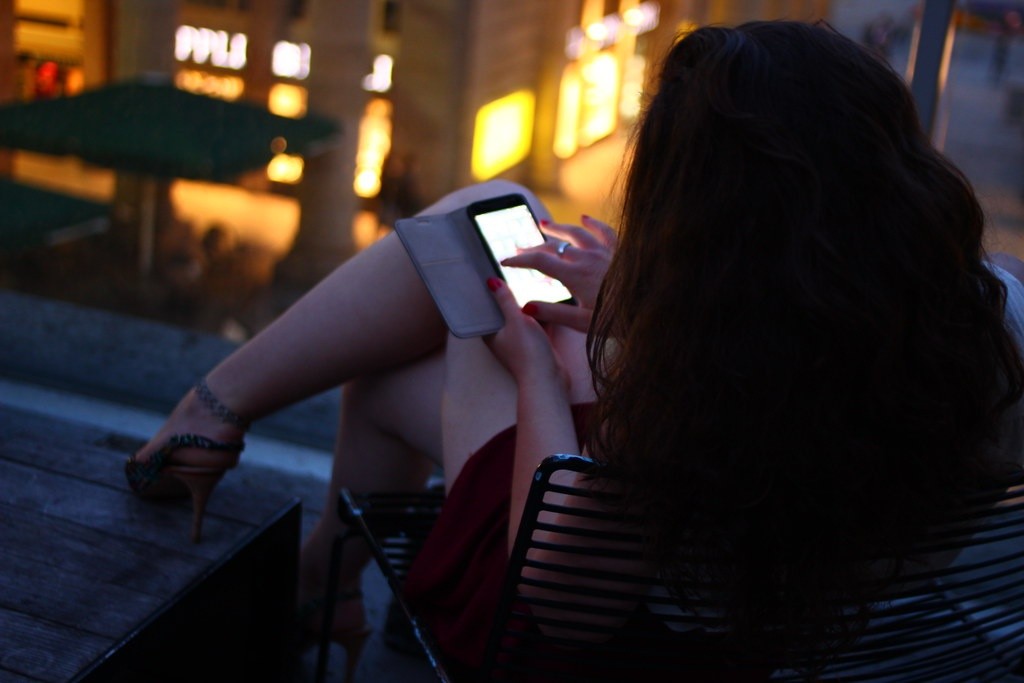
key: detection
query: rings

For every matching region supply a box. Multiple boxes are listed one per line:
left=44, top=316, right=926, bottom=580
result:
left=558, top=241, right=570, bottom=254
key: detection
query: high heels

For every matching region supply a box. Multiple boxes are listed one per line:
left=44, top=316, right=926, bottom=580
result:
left=302, top=591, right=373, bottom=679
left=124, top=434, right=247, bottom=543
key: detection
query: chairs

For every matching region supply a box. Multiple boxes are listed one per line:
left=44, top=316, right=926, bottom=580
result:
left=314, top=453, right=1024, bottom=683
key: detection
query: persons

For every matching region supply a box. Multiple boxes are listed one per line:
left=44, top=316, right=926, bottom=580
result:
left=125, top=20, right=1024, bottom=683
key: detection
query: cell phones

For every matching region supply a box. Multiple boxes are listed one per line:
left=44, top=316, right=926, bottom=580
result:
left=468, top=192, right=579, bottom=311
